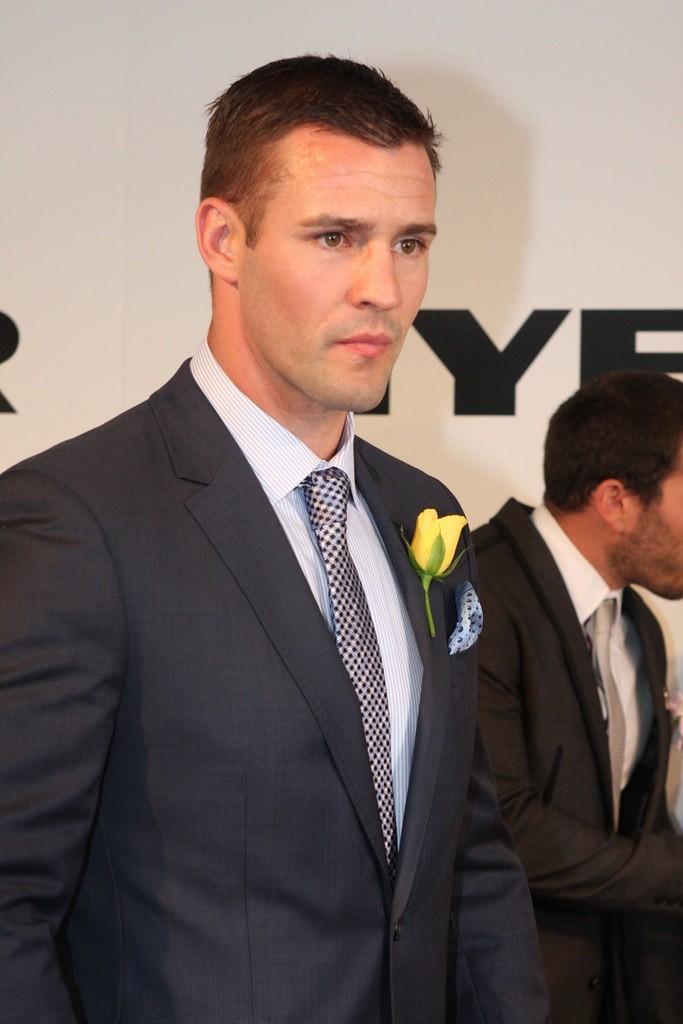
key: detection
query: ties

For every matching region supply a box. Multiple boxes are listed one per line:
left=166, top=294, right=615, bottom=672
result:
left=591, top=599, right=627, bottom=833
left=295, top=466, right=401, bottom=897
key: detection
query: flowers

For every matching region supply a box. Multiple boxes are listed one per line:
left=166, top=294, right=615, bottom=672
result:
left=663, top=689, right=683, bottom=751
left=398, top=509, right=468, bottom=639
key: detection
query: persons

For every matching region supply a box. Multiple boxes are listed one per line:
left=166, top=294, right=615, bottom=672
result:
left=471, top=370, right=683, bottom=1023
left=0, top=52, right=552, bottom=1023
